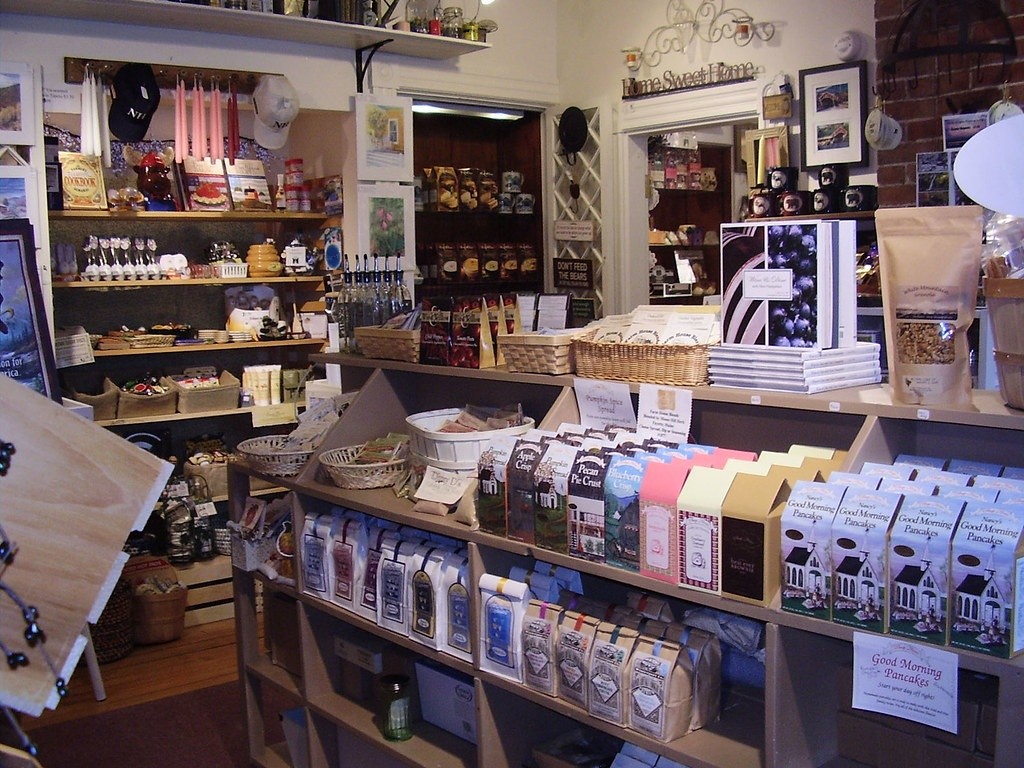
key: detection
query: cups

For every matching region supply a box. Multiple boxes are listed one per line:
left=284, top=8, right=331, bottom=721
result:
left=498, top=193, right=516, bottom=213
left=516, top=193, right=535, bottom=213
left=285, top=158, right=312, bottom=212
left=502, top=171, right=524, bottom=193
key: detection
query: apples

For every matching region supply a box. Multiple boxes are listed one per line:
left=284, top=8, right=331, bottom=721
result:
left=452, top=297, right=516, bottom=367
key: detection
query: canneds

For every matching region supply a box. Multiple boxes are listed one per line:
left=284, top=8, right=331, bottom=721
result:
left=378, top=673, right=412, bottom=742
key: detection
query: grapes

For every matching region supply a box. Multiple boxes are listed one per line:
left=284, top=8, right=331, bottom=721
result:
left=766, top=225, right=818, bottom=348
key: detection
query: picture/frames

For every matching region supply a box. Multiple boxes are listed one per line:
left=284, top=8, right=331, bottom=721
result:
left=800, top=60, right=869, bottom=171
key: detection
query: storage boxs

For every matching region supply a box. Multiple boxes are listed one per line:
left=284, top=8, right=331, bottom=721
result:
left=720, top=221, right=856, bottom=349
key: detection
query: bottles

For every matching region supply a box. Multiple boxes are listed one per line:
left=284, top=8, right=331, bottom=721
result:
left=153, top=575, right=167, bottom=592
left=442, top=6, right=464, bottom=38
left=677, top=225, right=697, bottom=245
left=350, top=255, right=363, bottom=355
left=406, top=0, right=428, bottom=34
left=418, top=242, right=538, bottom=282
left=372, top=252, right=383, bottom=327
left=338, top=255, right=355, bottom=354
left=144, top=575, right=152, bottom=584
left=383, top=253, right=393, bottom=325
left=275, top=174, right=286, bottom=211
left=362, top=254, right=373, bottom=327
left=165, top=577, right=174, bottom=587
left=392, top=252, right=412, bottom=318
left=415, top=167, right=499, bottom=213
left=379, top=675, right=415, bottom=742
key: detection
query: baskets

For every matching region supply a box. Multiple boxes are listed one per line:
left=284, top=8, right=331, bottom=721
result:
left=319, top=444, right=409, bottom=489
left=115, top=334, right=177, bottom=349
left=404, top=405, right=536, bottom=504
left=237, top=434, right=318, bottom=477
left=215, top=528, right=232, bottom=556
left=78, top=576, right=132, bottom=666
left=496, top=328, right=574, bottom=375
left=216, top=264, right=249, bottom=278
left=570, top=326, right=720, bottom=386
left=353, top=326, right=421, bottom=364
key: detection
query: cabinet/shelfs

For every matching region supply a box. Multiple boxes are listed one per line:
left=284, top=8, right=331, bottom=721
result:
left=229, top=353, right=1024, bottom=768
left=648, top=137, right=731, bottom=305
left=409, top=88, right=555, bottom=309
left=44, top=139, right=329, bottom=559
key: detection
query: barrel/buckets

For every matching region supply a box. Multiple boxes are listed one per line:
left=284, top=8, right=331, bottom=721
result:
left=405, top=405, right=534, bottom=508
left=982, top=276, right=1024, bottom=409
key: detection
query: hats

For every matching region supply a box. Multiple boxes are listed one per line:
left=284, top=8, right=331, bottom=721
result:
left=109, top=62, right=161, bottom=143
left=252, top=74, right=299, bottom=151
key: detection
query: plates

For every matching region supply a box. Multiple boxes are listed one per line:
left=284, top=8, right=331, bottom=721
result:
left=198, top=329, right=253, bottom=341
